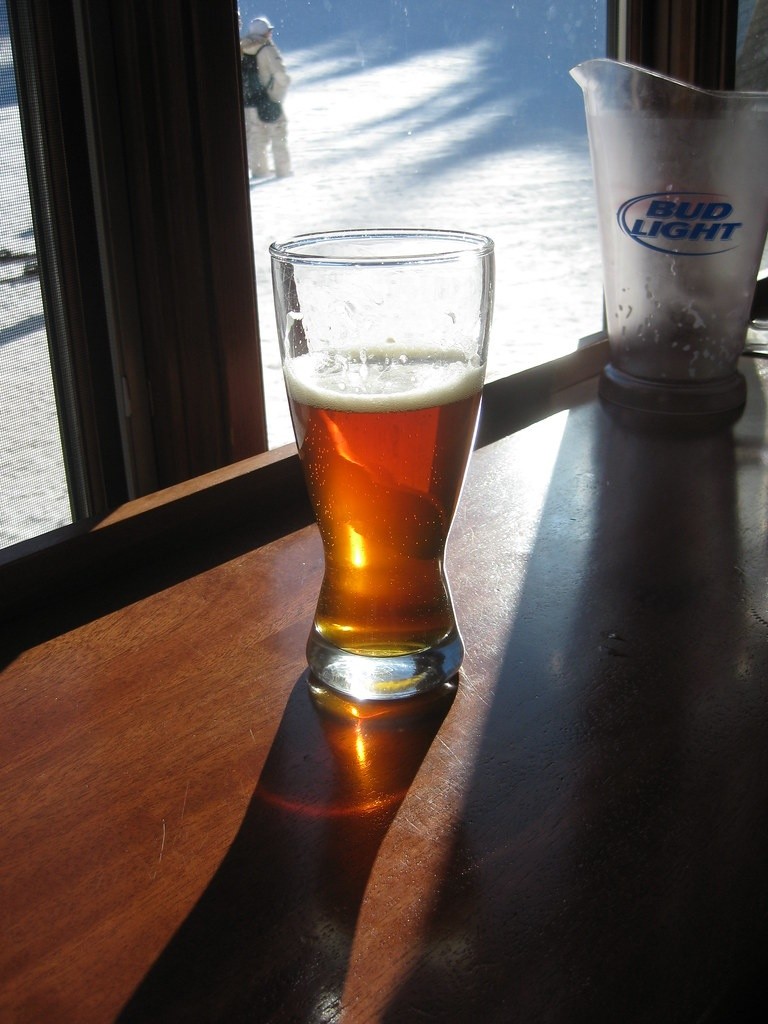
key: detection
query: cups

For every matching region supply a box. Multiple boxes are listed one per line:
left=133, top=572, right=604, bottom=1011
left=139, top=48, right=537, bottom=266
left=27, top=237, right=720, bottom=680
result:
left=569, top=58, right=768, bottom=430
left=268, top=231, right=496, bottom=699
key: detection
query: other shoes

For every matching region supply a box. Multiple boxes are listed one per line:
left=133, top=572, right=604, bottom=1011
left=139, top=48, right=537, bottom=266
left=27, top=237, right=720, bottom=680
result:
left=252, top=170, right=274, bottom=178
left=276, top=170, right=295, bottom=178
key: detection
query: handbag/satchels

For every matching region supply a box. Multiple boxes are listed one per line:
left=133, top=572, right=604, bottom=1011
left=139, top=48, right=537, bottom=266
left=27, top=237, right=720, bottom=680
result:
left=256, top=100, right=281, bottom=123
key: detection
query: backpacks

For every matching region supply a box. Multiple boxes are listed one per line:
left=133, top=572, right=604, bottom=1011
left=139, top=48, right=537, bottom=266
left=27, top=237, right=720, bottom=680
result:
left=240, top=42, right=274, bottom=107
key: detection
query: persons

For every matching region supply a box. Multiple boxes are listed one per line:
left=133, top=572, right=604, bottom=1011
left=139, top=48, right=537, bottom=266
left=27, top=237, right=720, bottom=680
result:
left=239, top=16, right=295, bottom=179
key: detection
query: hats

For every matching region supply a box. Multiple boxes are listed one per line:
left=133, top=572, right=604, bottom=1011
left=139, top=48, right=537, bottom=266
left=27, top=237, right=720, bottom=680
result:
left=249, top=17, right=275, bottom=36
